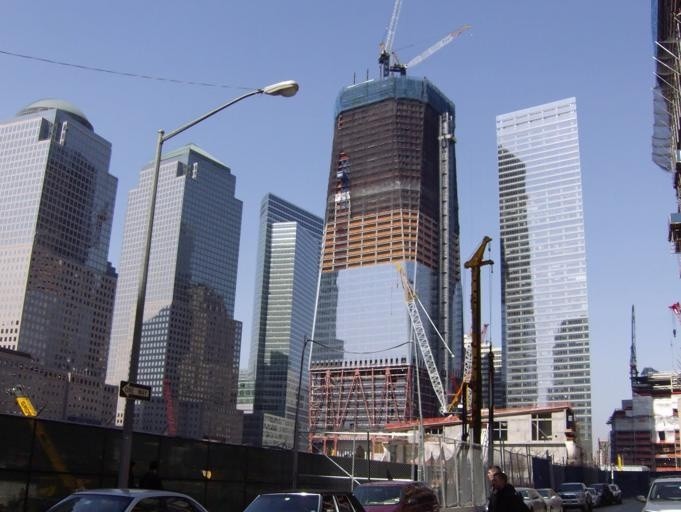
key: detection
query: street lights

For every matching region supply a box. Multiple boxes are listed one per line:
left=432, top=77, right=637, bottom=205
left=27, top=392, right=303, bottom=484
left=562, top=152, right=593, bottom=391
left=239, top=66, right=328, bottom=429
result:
left=117, top=79, right=298, bottom=489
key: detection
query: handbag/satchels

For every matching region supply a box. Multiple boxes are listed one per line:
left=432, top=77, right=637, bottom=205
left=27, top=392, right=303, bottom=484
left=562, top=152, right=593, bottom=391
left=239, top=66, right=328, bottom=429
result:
left=516, top=490, right=530, bottom=512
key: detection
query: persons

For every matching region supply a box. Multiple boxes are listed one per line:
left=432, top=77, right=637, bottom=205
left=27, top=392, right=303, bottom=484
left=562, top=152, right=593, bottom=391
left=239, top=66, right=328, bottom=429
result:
left=485, top=465, right=530, bottom=512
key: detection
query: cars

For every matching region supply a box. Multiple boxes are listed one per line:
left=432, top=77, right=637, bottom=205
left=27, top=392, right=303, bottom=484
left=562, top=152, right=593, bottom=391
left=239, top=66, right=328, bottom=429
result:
left=242, top=491, right=362, bottom=512
left=353, top=480, right=438, bottom=512
left=44, top=488, right=207, bottom=512
left=635, top=475, right=680, bottom=510
left=512, top=481, right=621, bottom=512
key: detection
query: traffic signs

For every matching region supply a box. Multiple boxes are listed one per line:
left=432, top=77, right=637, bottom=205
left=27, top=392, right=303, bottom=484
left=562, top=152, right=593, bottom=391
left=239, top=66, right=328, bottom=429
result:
left=118, top=381, right=152, bottom=403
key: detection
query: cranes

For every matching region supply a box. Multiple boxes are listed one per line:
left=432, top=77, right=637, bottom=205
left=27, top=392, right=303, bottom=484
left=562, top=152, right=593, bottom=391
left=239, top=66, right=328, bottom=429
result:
left=377, top=2, right=471, bottom=76
left=393, top=261, right=474, bottom=415
